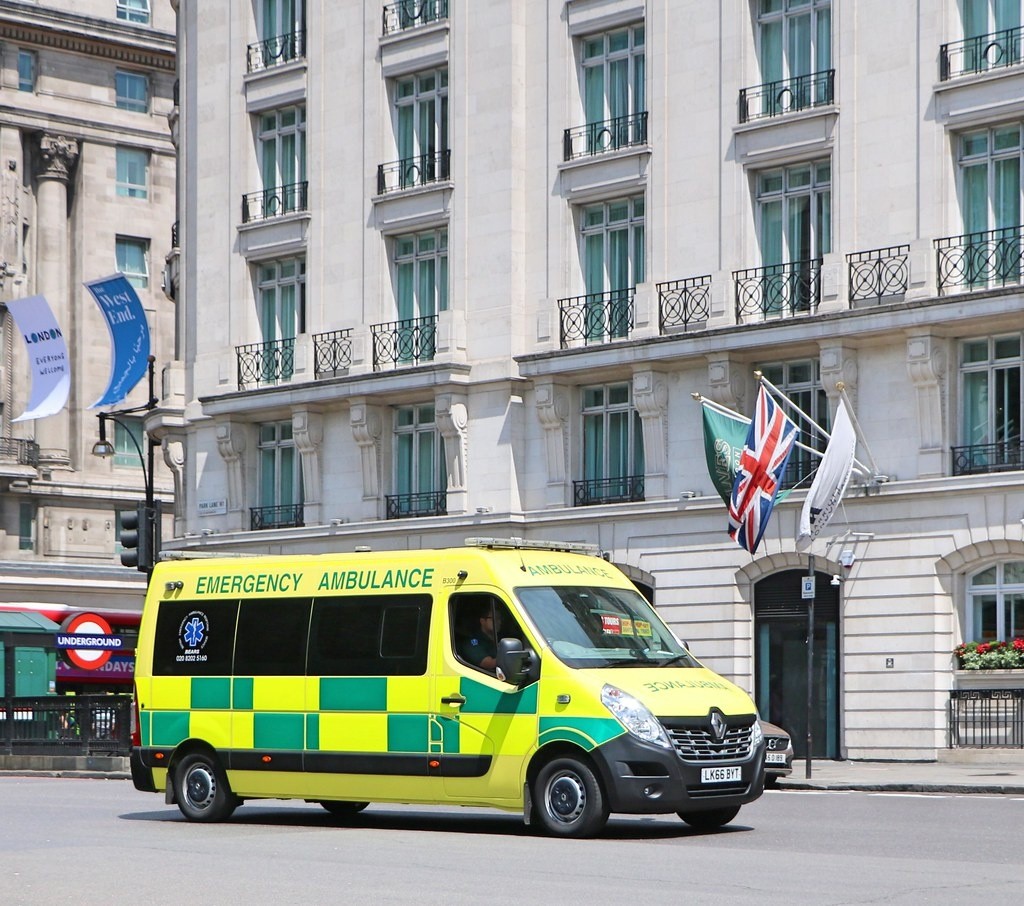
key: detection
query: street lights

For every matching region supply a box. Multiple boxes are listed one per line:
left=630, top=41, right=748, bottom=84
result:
left=92, top=355, right=160, bottom=578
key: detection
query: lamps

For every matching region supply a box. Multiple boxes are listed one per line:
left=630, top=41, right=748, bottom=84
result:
left=831, top=574, right=845, bottom=585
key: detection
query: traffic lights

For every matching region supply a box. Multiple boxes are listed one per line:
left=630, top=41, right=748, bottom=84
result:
left=118, top=499, right=153, bottom=572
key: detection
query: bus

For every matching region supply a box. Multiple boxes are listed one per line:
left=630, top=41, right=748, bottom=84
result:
left=0, top=602, right=143, bottom=695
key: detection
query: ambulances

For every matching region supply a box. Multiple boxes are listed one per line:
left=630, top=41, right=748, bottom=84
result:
left=131, top=537, right=767, bottom=839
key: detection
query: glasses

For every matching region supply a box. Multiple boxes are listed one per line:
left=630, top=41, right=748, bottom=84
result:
left=482, top=617, right=501, bottom=621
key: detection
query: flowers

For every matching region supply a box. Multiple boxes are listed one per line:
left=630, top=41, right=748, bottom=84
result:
left=952, top=635, right=1024, bottom=671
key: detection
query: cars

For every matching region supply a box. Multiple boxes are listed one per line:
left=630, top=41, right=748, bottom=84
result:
left=757, top=719, right=794, bottom=789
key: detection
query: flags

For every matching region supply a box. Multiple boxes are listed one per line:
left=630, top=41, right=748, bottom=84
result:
left=701, top=403, right=793, bottom=512
left=796, top=394, right=857, bottom=552
left=728, top=382, right=801, bottom=555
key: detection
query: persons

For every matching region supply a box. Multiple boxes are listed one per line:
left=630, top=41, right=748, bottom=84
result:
left=459, top=603, right=502, bottom=673
